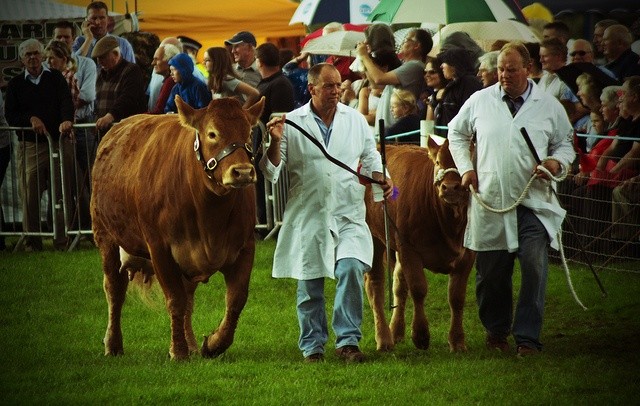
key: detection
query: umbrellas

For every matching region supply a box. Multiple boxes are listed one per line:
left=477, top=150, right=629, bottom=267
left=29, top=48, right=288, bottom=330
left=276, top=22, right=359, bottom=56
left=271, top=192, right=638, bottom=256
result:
left=287, top=0, right=379, bottom=29
left=432, top=20, right=542, bottom=48
left=392, top=27, right=436, bottom=54
left=367, top=0, right=517, bottom=26
left=553, top=62, right=622, bottom=110
left=299, top=23, right=368, bottom=47
left=300, top=30, right=368, bottom=61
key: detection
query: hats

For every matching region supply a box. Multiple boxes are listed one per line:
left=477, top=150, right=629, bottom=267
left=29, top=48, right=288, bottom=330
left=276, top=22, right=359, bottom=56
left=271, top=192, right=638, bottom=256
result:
left=177, top=36, right=202, bottom=51
left=91, top=34, right=120, bottom=59
left=224, top=32, right=256, bottom=47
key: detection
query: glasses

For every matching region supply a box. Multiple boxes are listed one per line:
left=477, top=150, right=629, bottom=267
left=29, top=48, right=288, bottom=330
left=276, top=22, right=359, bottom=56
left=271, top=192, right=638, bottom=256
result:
left=202, top=58, right=211, bottom=61
left=569, top=50, right=592, bottom=56
left=402, top=36, right=416, bottom=43
left=423, top=70, right=435, bottom=75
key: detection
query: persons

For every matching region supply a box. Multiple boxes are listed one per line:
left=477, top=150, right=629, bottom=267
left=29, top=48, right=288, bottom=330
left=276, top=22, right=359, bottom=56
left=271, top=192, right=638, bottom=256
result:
left=385, top=87, right=420, bottom=142
left=423, top=57, right=448, bottom=121
left=476, top=51, right=500, bottom=87
left=45, top=39, right=80, bottom=174
left=4, top=39, right=79, bottom=252
left=574, top=88, right=630, bottom=176
left=258, top=62, right=395, bottom=363
left=596, top=76, right=640, bottom=173
left=277, top=37, right=287, bottom=48
left=586, top=9, right=604, bottom=41
left=340, top=55, right=371, bottom=109
left=143, top=36, right=183, bottom=95
left=203, top=47, right=260, bottom=110
left=538, top=38, right=570, bottom=98
left=630, top=8, right=640, bottom=19
left=563, top=33, right=590, bottom=61
left=177, top=35, right=207, bottom=85
left=630, top=21, right=640, bottom=56
left=558, top=38, right=617, bottom=123
left=164, top=53, right=212, bottom=113
left=600, top=24, right=640, bottom=82
left=587, top=108, right=608, bottom=148
left=586, top=8, right=587, bottom=9
left=90, top=34, right=143, bottom=144
left=573, top=71, right=603, bottom=129
left=41, top=19, right=98, bottom=229
left=282, top=53, right=330, bottom=109
left=279, top=49, right=295, bottom=67
left=357, top=79, right=385, bottom=126
left=0, top=89, right=13, bottom=251
left=446, top=40, right=577, bottom=361
left=325, top=55, right=358, bottom=82
left=143, top=43, right=180, bottom=114
left=610, top=174, right=640, bottom=240
left=355, top=28, right=433, bottom=143
left=560, top=30, right=578, bottom=46
left=539, top=21, right=569, bottom=43
left=609, top=8, right=628, bottom=27
left=434, top=51, right=484, bottom=138
left=224, top=30, right=262, bottom=156
left=488, top=39, right=510, bottom=52
left=573, top=86, right=622, bottom=186
left=72, top=1, right=136, bottom=78
left=254, top=42, right=295, bottom=238
left=592, top=18, right=619, bottom=66
left=522, top=42, right=543, bottom=85
left=323, top=21, right=346, bottom=36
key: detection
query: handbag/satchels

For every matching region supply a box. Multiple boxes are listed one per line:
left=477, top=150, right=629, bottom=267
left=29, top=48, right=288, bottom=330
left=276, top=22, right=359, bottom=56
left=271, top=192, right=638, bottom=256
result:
left=54, top=138, right=85, bottom=174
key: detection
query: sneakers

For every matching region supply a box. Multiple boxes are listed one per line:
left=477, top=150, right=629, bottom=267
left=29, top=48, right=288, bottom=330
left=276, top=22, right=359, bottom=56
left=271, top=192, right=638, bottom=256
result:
left=305, top=353, right=324, bottom=362
left=517, top=346, right=534, bottom=357
left=486, top=337, right=507, bottom=353
left=336, top=345, right=367, bottom=363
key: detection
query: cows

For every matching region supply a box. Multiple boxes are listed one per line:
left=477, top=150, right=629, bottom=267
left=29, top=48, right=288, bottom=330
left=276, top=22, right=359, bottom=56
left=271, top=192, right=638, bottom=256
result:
left=363, top=135, right=475, bottom=354
left=89, top=94, right=268, bottom=363
